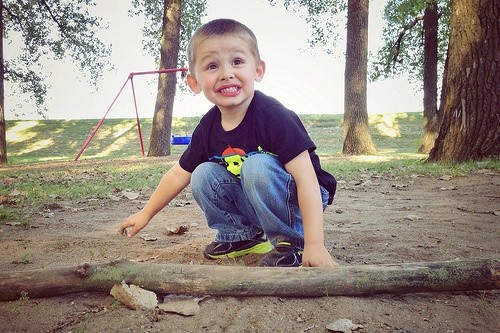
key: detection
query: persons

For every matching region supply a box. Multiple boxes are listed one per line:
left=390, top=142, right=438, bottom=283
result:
left=119, top=19, right=339, bottom=268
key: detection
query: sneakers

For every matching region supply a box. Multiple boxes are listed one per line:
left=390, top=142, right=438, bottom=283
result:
left=204, top=229, right=273, bottom=259
left=259, top=241, right=304, bottom=267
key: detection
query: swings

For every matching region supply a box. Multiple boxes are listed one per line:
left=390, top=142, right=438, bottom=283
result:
left=167, top=71, right=194, bottom=146
